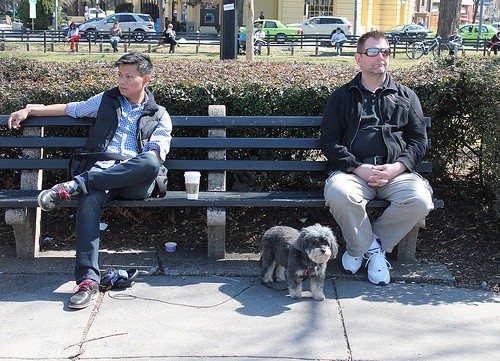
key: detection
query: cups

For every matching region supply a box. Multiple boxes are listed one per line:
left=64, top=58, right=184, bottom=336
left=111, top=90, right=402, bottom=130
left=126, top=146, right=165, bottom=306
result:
left=184, top=171, right=201, bottom=199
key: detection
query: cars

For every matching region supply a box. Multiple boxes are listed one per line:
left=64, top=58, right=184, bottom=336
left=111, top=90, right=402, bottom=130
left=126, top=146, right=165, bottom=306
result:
left=84, top=8, right=107, bottom=21
left=459, top=24, right=500, bottom=49
left=238, top=18, right=305, bottom=44
left=61, top=16, right=118, bottom=39
left=383, top=23, right=427, bottom=44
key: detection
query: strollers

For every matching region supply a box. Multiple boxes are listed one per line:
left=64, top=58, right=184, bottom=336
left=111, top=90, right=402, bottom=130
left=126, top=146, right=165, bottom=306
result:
left=240, top=31, right=261, bottom=57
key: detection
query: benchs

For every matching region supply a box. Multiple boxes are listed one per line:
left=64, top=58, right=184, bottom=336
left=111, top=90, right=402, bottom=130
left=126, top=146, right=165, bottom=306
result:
left=0, top=104, right=444, bottom=262
left=260, top=31, right=492, bottom=59
left=0, top=29, right=220, bottom=54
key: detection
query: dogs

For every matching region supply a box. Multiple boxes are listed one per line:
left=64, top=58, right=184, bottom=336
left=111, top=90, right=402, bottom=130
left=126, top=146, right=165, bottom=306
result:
left=255, top=222, right=341, bottom=300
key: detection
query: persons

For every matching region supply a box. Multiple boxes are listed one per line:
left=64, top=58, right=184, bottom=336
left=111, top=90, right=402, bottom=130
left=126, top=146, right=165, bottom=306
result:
left=331, top=28, right=346, bottom=56
left=447, top=28, right=462, bottom=58
left=237, top=25, right=242, bottom=55
left=66, top=22, right=81, bottom=52
left=258, top=11, right=265, bottom=20
left=417, top=22, right=424, bottom=27
left=320, top=31, right=434, bottom=286
left=487, top=25, right=500, bottom=55
left=7, top=51, right=172, bottom=309
left=468, top=27, right=472, bottom=32
left=164, top=24, right=180, bottom=53
left=109, top=21, right=122, bottom=52
left=254, top=24, right=265, bottom=55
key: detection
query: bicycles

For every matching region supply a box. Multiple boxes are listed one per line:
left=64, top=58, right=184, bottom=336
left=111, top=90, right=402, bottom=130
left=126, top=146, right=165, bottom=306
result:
left=405, top=33, right=450, bottom=60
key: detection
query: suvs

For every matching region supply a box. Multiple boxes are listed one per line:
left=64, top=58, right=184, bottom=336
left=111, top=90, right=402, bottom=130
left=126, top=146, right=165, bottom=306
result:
left=285, top=15, right=354, bottom=47
left=79, top=12, right=155, bottom=42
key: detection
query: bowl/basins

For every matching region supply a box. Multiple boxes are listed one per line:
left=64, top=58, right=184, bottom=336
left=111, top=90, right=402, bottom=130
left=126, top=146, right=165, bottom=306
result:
left=165, top=242, right=177, bottom=252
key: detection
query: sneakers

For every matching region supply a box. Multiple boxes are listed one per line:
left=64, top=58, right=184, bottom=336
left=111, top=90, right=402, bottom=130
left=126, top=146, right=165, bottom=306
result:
left=341, top=250, right=364, bottom=275
left=363, top=247, right=392, bottom=286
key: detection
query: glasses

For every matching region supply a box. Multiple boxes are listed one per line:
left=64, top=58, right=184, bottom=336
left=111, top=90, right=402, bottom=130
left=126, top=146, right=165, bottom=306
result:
left=359, top=47, right=391, bottom=57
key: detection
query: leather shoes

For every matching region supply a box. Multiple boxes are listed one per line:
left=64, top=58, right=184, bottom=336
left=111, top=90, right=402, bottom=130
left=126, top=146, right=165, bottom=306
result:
left=68, top=279, right=99, bottom=308
left=38, top=184, right=73, bottom=212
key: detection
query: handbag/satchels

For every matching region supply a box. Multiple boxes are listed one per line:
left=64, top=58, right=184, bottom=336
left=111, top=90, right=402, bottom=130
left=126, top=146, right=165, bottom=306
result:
left=67, top=148, right=84, bottom=181
